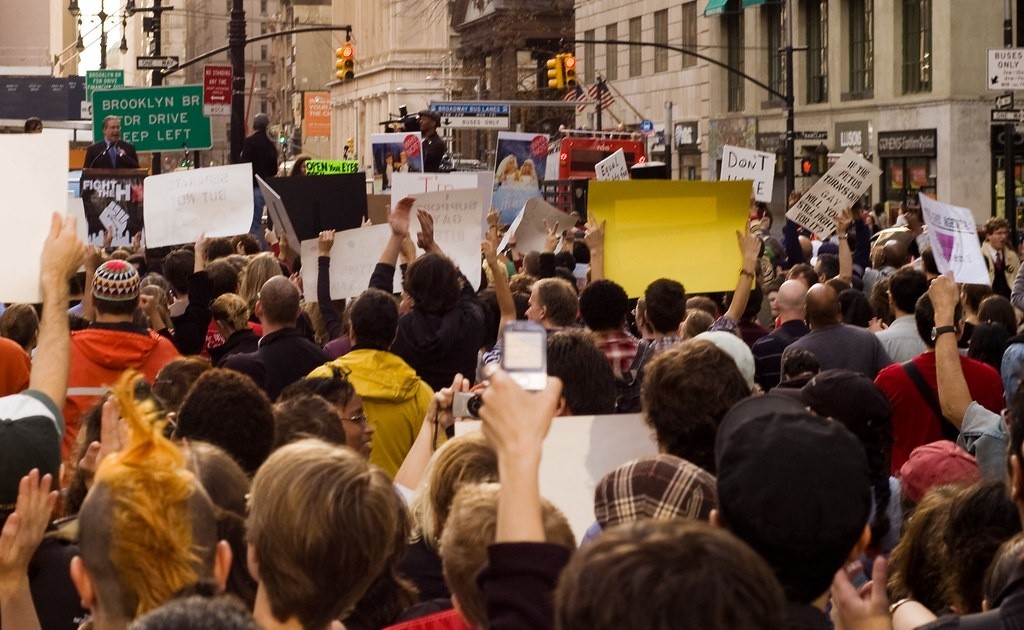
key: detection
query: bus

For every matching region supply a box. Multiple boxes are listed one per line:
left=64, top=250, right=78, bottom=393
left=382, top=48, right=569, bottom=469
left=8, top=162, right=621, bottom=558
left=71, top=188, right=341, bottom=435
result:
left=543, top=130, right=647, bottom=232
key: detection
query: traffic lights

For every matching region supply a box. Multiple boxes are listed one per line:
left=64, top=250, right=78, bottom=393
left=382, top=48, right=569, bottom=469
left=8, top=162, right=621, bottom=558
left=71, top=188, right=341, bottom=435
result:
left=801, top=158, right=818, bottom=176
left=564, top=56, right=577, bottom=89
left=547, top=57, right=564, bottom=89
left=342, top=46, right=355, bottom=81
left=347, top=137, right=354, bottom=153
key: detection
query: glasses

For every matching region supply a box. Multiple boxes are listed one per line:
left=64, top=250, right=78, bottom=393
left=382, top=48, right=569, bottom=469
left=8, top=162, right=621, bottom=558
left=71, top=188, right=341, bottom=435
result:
left=339, top=415, right=368, bottom=425
left=631, top=309, right=636, bottom=315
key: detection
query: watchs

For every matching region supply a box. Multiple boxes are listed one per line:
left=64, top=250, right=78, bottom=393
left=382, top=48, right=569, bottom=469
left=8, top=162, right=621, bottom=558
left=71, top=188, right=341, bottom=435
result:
left=930, top=326, right=958, bottom=340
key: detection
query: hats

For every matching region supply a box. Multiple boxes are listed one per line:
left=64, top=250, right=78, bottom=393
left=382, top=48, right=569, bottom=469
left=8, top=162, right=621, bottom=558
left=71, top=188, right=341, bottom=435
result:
left=1001, top=343, right=1024, bottom=410
left=900, top=439, right=982, bottom=505
left=0, top=390, right=65, bottom=508
left=91, top=259, right=140, bottom=302
left=594, top=452, right=717, bottom=530
left=418, top=109, right=441, bottom=127
left=714, top=394, right=876, bottom=572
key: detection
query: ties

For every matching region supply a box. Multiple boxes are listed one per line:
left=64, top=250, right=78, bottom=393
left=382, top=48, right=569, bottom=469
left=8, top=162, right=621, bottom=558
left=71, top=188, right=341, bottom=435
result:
left=110, top=147, right=116, bottom=169
left=996, top=252, right=1001, bottom=270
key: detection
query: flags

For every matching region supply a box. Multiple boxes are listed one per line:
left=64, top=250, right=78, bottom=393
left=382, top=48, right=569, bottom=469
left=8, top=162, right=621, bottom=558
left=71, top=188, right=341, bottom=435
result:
left=561, top=83, right=587, bottom=111
left=588, top=75, right=615, bottom=115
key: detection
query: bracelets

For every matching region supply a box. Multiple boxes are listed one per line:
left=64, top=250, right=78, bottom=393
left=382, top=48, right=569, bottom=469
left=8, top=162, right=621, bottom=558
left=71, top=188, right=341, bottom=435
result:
left=837, top=232, right=849, bottom=239
left=739, top=269, right=755, bottom=279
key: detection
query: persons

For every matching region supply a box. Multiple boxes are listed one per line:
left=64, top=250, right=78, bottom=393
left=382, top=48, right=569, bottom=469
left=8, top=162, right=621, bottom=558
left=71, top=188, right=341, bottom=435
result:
left=238, top=113, right=278, bottom=233
left=381, top=150, right=421, bottom=190
left=83, top=116, right=139, bottom=168
left=391, top=110, right=447, bottom=172
left=24, top=117, right=43, bottom=133
left=290, top=156, right=311, bottom=175
left=494, top=155, right=540, bottom=185
left=0, top=191, right=1024, bottom=630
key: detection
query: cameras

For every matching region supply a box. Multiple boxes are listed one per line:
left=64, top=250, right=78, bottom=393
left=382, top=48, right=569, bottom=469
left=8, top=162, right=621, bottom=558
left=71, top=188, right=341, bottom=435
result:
left=452, top=392, right=482, bottom=419
left=500, top=320, right=548, bottom=390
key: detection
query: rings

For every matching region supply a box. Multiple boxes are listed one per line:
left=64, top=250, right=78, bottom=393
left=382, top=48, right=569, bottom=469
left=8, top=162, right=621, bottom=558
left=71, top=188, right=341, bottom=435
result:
left=584, top=229, right=589, bottom=234
left=554, top=233, right=562, bottom=240
left=481, top=362, right=501, bottom=379
left=319, top=232, right=322, bottom=236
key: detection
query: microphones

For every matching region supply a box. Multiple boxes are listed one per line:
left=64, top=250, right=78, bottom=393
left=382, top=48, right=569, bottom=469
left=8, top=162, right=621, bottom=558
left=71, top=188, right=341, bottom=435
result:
left=103, top=141, right=115, bottom=155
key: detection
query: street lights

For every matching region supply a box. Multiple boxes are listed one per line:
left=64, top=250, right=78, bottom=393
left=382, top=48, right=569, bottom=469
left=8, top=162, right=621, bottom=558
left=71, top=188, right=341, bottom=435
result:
left=76, top=0, right=128, bottom=71
left=396, top=87, right=453, bottom=155
left=425, top=76, right=481, bottom=161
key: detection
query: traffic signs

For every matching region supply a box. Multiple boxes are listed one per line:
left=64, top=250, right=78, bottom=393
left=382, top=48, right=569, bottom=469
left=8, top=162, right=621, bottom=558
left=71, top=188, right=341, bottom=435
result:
left=990, top=109, right=1024, bottom=124
left=987, top=48, right=1024, bottom=90
left=137, top=56, right=179, bottom=71
left=995, top=92, right=1014, bottom=111
left=148, top=39, right=156, bottom=56
